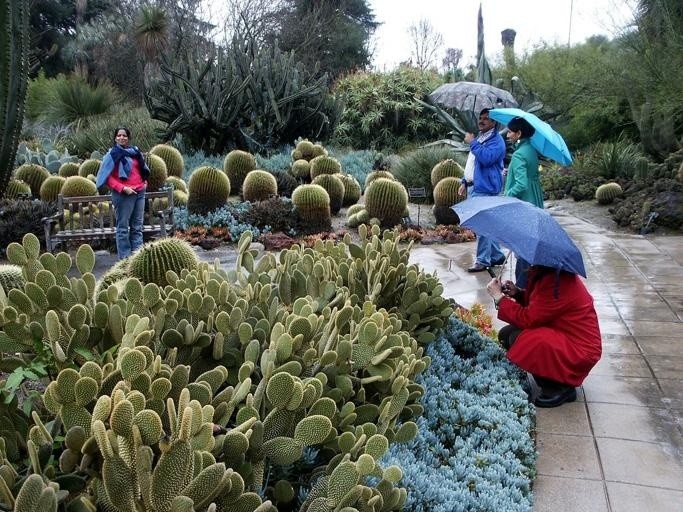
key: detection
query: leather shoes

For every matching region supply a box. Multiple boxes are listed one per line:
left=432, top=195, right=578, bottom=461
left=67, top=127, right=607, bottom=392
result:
left=534, top=388, right=577, bottom=408
left=490, top=256, right=506, bottom=265
left=467, top=262, right=490, bottom=272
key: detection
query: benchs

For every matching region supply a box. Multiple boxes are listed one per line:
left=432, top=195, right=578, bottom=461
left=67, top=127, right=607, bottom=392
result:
left=41, top=187, right=176, bottom=253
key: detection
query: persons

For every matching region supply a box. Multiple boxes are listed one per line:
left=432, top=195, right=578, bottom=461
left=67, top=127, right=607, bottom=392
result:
left=501, top=116, right=544, bottom=287
left=457, top=108, right=507, bottom=273
left=97, top=127, right=150, bottom=260
left=487, top=265, right=601, bottom=408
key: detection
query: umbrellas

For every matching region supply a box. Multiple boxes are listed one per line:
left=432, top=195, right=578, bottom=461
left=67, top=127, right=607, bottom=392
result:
left=429, top=81, right=519, bottom=133
left=488, top=108, right=573, bottom=168
left=448, top=196, right=587, bottom=280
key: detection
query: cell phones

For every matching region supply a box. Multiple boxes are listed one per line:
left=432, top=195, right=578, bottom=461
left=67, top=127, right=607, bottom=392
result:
left=486, top=266, right=507, bottom=290
